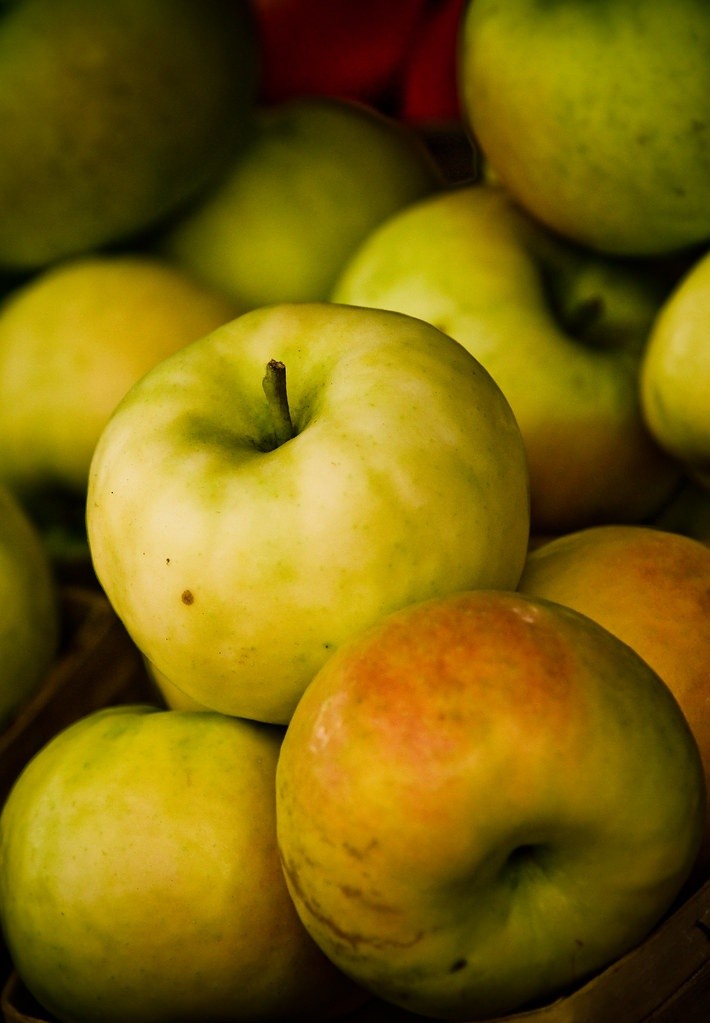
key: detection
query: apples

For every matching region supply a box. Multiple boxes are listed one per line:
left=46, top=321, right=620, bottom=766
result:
left=0, top=0, right=710, bottom=1023
